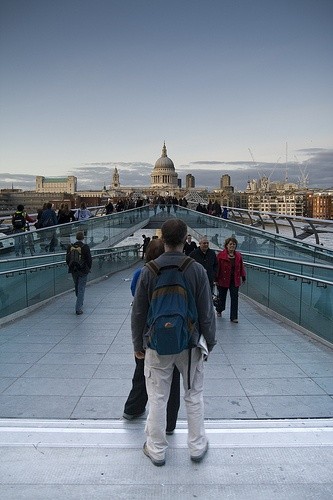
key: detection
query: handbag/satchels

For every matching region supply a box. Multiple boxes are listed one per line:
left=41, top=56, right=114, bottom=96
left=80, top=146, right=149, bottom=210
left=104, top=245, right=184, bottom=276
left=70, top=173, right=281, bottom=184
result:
left=212, top=293, right=223, bottom=307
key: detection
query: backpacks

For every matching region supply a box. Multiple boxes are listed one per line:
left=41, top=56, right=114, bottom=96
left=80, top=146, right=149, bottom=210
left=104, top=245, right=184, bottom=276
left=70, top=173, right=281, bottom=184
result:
left=12, top=212, right=25, bottom=230
left=145, top=256, right=201, bottom=389
left=68, top=242, right=87, bottom=273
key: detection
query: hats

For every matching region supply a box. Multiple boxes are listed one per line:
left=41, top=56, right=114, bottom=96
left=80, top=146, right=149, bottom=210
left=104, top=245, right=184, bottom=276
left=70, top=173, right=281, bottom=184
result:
left=17, top=205, right=24, bottom=211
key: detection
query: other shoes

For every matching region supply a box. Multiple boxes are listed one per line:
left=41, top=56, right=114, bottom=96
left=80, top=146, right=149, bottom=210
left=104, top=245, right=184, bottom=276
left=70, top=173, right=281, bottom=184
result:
left=166, top=430, right=174, bottom=435
left=143, top=441, right=166, bottom=467
left=123, top=413, right=136, bottom=420
left=190, top=441, right=208, bottom=462
left=217, top=312, right=222, bottom=317
left=230, top=318, right=238, bottom=323
left=76, top=311, right=83, bottom=315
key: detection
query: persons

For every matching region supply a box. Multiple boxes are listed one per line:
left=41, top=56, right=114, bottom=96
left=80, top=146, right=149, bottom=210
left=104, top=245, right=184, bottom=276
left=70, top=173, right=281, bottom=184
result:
left=130, top=220, right=216, bottom=467
left=44, top=233, right=109, bottom=254
left=123, top=240, right=180, bottom=436
left=105, top=193, right=229, bottom=228
left=210, top=232, right=277, bottom=255
left=12, top=204, right=36, bottom=257
left=66, top=233, right=92, bottom=314
left=139, top=233, right=197, bottom=262
left=214, top=238, right=247, bottom=324
left=33, top=200, right=95, bottom=253
left=189, top=237, right=216, bottom=294
